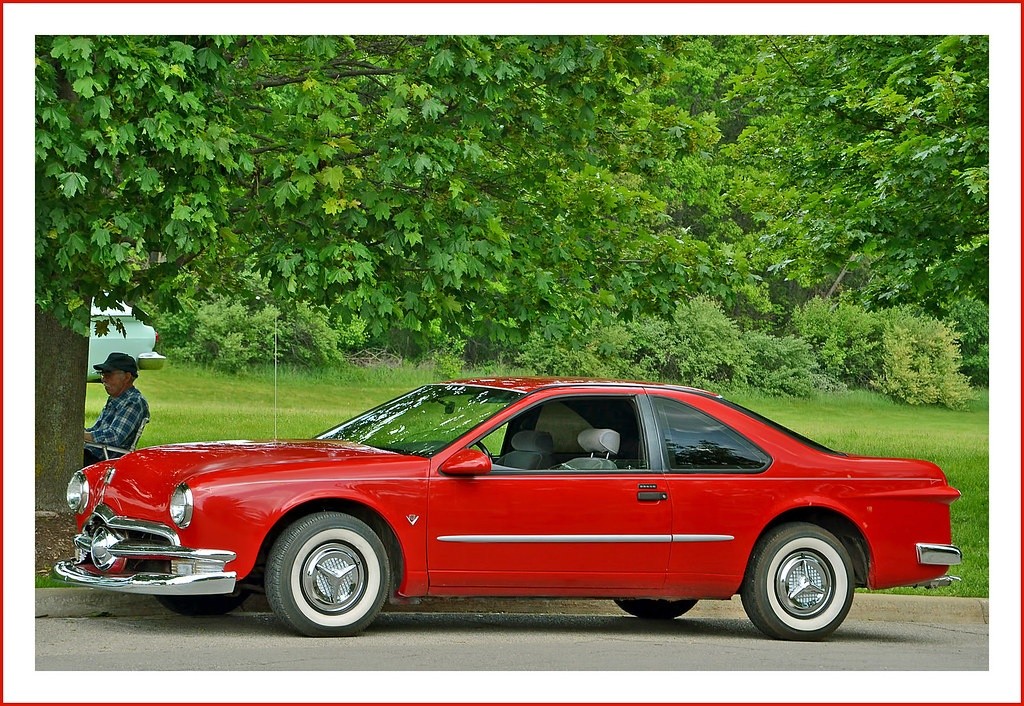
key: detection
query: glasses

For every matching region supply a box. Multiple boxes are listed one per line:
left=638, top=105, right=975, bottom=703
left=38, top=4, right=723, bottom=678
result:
left=96, top=372, right=124, bottom=379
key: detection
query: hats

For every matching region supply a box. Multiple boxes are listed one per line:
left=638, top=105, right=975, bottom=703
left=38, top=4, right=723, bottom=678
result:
left=92, top=352, right=139, bottom=378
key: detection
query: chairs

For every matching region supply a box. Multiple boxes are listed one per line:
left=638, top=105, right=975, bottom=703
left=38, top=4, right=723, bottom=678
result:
left=558, top=428, right=620, bottom=470
left=85, top=411, right=150, bottom=460
left=495, top=431, right=556, bottom=470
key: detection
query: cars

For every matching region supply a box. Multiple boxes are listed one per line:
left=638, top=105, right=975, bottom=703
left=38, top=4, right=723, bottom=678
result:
left=54, top=377, right=963, bottom=641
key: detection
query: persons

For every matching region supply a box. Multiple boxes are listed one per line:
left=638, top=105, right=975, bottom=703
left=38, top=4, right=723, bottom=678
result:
left=84, top=352, right=150, bottom=468
